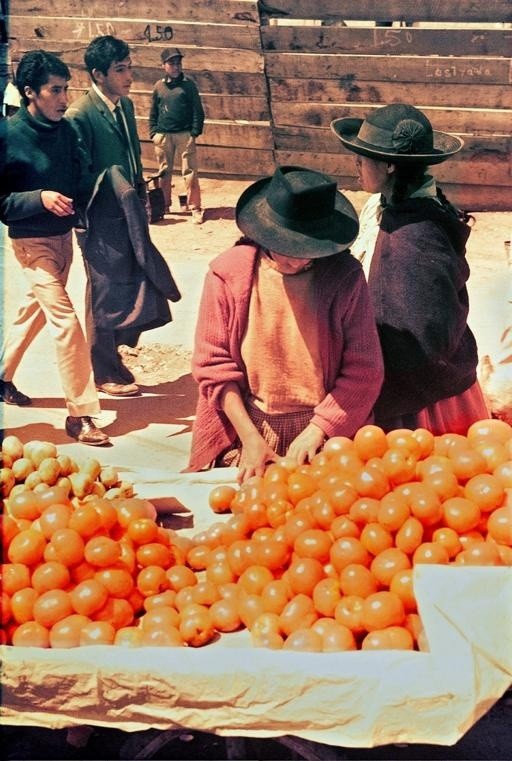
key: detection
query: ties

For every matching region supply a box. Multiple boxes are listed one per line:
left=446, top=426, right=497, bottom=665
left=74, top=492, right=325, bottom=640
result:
left=114, top=106, right=135, bottom=176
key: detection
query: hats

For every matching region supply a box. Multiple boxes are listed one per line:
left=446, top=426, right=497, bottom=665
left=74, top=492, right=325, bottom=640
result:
left=161, top=48, right=183, bottom=63
left=235, top=166, right=359, bottom=259
left=330, top=104, right=465, bottom=165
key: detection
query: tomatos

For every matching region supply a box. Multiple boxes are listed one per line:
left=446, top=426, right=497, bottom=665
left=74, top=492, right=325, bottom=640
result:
left=0, top=418, right=512, bottom=652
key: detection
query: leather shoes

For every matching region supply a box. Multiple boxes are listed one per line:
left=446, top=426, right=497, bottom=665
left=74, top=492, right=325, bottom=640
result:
left=0, top=379, right=32, bottom=405
left=95, top=376, right=139, bottom=396
left=66, top=417, right=110, bottom=445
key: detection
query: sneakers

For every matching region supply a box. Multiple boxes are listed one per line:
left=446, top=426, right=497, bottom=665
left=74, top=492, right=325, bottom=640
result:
left=191, top=208, right=204, bottom=224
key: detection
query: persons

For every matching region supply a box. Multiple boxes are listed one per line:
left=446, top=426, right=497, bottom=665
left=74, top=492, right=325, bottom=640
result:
left=148, top=48, right=205, bottom=223
left=330, top=104, right=491, bottom=438
left=0, top=50, right=110, bottom=446
left=181, top=167, right=387, bottom=487
left=63, top=36, right=151, bottom=397
